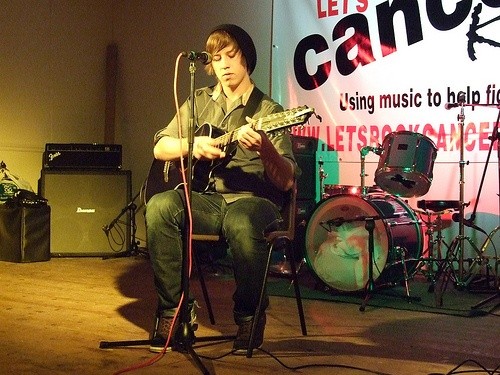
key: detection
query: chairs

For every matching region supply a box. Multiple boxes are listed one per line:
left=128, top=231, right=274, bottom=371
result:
left=156, top=172, right=308, bottom=359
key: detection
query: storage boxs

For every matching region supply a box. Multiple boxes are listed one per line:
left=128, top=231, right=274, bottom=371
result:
left=44, top=142, right=122, bottom=170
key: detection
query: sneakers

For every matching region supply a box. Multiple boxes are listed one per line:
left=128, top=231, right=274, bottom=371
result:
left=233, top=311, right=266, bottom=354
left=150, top=316, right=179, bottom=353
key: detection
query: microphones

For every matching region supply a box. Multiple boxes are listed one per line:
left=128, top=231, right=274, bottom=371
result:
left=452, top=213, right=486, bottom=234
left=321, top=217, right=344, bottom=226
left=103, top=225, right=111, bottom=231
left=182, top=51, right=213, bottom=64
left=445, top=102, right=459, bottom=110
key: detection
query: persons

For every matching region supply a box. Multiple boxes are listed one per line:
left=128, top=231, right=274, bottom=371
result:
left=144, top=25, right=298, bottom=352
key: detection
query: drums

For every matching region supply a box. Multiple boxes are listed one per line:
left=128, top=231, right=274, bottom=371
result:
left=324, top=184, right=373, bottom=197
left=304, top=192, right=424, bottom=293
left=374, top=130, right=439, bottom=198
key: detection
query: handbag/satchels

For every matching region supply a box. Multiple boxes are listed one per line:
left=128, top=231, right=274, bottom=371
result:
left=5, top=188, right=48, bottom=206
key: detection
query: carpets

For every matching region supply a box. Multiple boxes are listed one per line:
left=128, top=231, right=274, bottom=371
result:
left=264, top=270, right=500, bottom=318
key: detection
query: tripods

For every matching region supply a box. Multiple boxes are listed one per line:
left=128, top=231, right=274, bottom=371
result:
left=100, top=60, right=236, bottom=375
left=329, top=103, right=499, bottom=314
left=103, top=186, right=151, bottom=259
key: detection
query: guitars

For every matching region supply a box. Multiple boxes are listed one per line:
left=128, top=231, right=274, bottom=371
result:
left=143, top=105, right=322, bottom=206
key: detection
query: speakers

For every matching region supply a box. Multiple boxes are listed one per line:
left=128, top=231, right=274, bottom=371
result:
left=291, top=135, right=340, bottom=258
left=40, top=169, right=132, bottom=256
left=0, top=206, right=50, bottom=263
